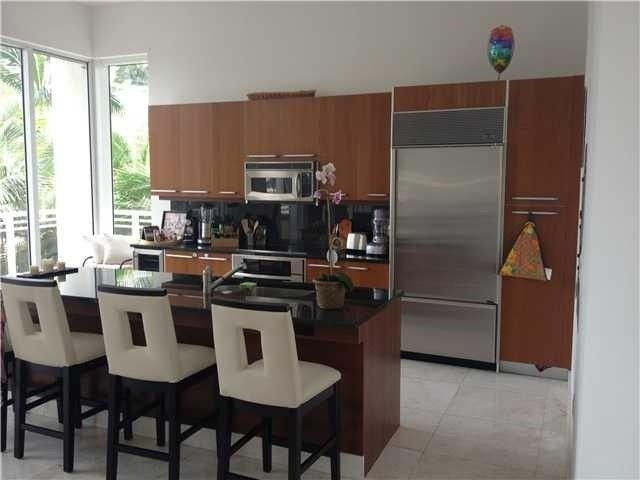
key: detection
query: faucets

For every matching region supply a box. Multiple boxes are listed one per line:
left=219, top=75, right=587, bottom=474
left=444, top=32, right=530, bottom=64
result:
left=201, top=262, right=247, bottom=292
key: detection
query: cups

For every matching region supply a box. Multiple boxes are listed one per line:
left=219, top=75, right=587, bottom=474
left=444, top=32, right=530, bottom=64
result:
left=30, top=259, right=65, bottom=275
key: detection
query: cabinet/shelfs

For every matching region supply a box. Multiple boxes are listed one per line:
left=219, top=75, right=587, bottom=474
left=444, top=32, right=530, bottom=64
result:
left=304, top=261, right=389, bottom=288
left=500, top=75, right=585, bottom=369
left=151, top=100, right=249, bottom=197
left=248, top=96, right=325, bottom=161
left=163, top=248, right=232, bottom=276
left=322, top=91, right=394, bottom=199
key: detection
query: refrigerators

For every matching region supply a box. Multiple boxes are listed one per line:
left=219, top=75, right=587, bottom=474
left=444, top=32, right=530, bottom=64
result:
left=386, top=104, right=505, bottom=367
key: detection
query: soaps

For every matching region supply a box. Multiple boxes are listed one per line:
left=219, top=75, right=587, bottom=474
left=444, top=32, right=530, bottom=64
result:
left=240, top=282, right=256, bottom=288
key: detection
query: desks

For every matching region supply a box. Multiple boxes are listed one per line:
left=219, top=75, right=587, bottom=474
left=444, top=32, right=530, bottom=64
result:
left=11, top=264, right=403, bottom=479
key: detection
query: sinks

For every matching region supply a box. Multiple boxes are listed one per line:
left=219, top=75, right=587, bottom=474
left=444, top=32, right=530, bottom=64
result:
left=236, top=286, right=314, bottom=302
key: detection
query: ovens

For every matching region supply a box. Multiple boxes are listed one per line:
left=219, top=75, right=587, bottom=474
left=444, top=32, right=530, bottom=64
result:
left=231, top=254, right=306, bottom=283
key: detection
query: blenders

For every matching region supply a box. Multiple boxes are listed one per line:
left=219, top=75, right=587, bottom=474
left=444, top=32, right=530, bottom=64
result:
left=197, top=204, right=215, bottom=245
left=365, top=208, right=389, bottom=258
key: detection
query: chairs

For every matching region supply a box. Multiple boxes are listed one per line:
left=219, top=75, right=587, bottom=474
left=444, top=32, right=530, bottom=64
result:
left=2, top=275, right=110, bottom=472
left=95, top=282, right=219, bottom=480
left=204, top=292, right=345, bottom=479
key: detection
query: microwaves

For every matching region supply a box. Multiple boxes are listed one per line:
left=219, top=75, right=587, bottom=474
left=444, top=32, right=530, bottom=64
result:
left=243, top=160, right=315, bottom=203
